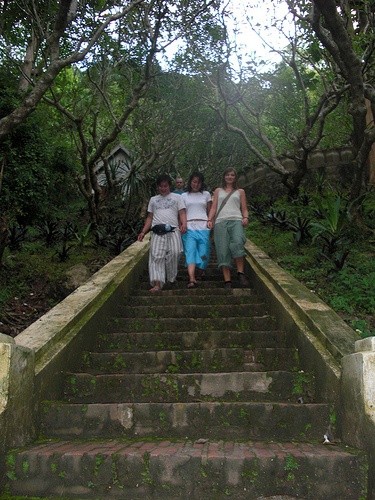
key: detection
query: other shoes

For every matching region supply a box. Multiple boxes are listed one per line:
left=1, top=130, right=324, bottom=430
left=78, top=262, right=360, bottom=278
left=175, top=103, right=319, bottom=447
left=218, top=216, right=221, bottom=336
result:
left=238, top=275, right=248, bottom=286
left=186, top=281, right=197, bottom=288
left=151, top=289, right=158, bottom=291
left=224, top=282, right=231, bottom=288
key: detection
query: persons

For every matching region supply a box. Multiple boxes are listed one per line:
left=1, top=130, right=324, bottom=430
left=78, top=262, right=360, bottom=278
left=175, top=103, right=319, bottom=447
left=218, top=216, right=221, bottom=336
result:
left=138, top=175, right=187, bottom=293
left=207, top=167, right=249, bottom=292
left=178, top=172, right=214, bottom=290
left=172, top=176, right=186, bottom=195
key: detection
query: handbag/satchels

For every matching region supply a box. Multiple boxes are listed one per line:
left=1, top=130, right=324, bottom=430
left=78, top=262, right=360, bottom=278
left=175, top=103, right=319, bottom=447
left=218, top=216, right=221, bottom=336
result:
left=151, top=224, right=176, bottom=235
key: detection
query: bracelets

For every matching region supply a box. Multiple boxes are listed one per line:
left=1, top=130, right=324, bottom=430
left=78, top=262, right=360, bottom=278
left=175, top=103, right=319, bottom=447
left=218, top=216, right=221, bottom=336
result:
left=243, top=217, right=249, bottom=219
left=208, top=220, right=212, bottom=222
left=141, top=231, right=144, bottom=233
